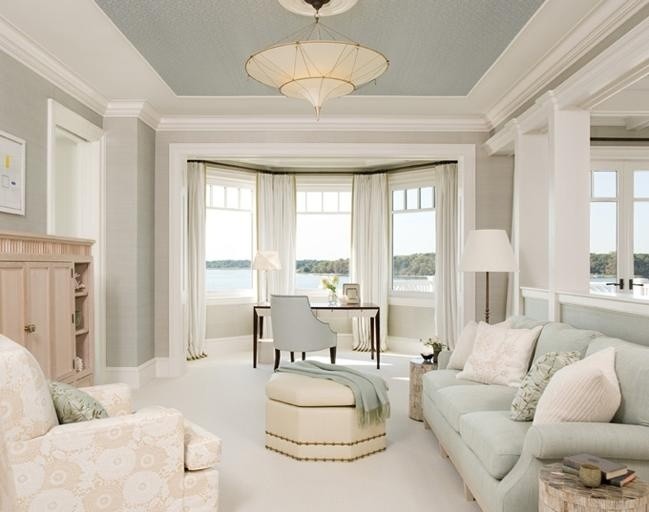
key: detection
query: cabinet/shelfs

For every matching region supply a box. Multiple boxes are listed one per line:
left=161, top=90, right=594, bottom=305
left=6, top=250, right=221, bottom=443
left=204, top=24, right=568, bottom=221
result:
left=0, top=233, right=95, bottom=387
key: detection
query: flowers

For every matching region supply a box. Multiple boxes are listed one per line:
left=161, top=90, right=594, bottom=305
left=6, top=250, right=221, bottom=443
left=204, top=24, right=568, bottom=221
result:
left=320, top=275, right=339, bottom=293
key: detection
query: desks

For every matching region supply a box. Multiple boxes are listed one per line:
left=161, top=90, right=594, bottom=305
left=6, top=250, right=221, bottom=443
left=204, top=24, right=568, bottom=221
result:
left=266, top=365, right=389, bottom=463
left=538, top=461, right=648, bottom=511
left=252, top=300, right=381, bottom=368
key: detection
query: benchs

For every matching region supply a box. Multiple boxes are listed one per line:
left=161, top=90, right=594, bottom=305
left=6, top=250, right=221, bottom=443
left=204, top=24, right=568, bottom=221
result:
left=409, top=359, right=438, bottom=422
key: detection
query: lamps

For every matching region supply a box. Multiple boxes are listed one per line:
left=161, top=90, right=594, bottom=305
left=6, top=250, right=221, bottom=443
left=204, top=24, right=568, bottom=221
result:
left=245, top=1, right=389, bottom=122
left=457, top=229, right=519, bottom=324
left=252, top=251, right=282, bottom=304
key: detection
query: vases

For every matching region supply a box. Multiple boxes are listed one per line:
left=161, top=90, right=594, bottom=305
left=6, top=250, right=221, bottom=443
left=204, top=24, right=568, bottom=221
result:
left=328, top=293, right=338, bottom=305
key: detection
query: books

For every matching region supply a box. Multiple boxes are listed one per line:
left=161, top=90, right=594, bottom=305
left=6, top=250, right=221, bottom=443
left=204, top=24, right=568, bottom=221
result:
left=564, top=454, right=630, bottom=481
left=560, top=464, right=637, bottom=486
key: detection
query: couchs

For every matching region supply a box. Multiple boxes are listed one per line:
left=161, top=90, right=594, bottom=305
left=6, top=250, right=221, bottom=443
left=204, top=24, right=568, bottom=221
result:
left=421, top=313, right=648, bottom=512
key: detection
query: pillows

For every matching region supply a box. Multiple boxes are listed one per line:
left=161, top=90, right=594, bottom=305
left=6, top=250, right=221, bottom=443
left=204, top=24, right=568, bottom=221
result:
left=444, top=319, right=621, bottom=425
left=46, top=374, right=109, bottom=426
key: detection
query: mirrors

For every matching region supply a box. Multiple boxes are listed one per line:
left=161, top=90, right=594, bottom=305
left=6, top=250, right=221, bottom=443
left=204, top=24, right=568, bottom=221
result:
left=588, top=74, right=649, bottom=304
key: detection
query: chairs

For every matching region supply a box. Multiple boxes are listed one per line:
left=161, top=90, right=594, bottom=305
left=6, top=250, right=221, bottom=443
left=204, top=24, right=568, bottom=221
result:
left=269, top=293, right=338, bottom=372
left=0, top=335, right=221, bottom=512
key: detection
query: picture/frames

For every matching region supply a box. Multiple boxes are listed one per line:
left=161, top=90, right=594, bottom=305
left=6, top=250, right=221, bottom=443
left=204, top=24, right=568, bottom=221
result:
left=0, top=129, right=27, bottom=217
left=342, top=283, right=361, bottom=305
